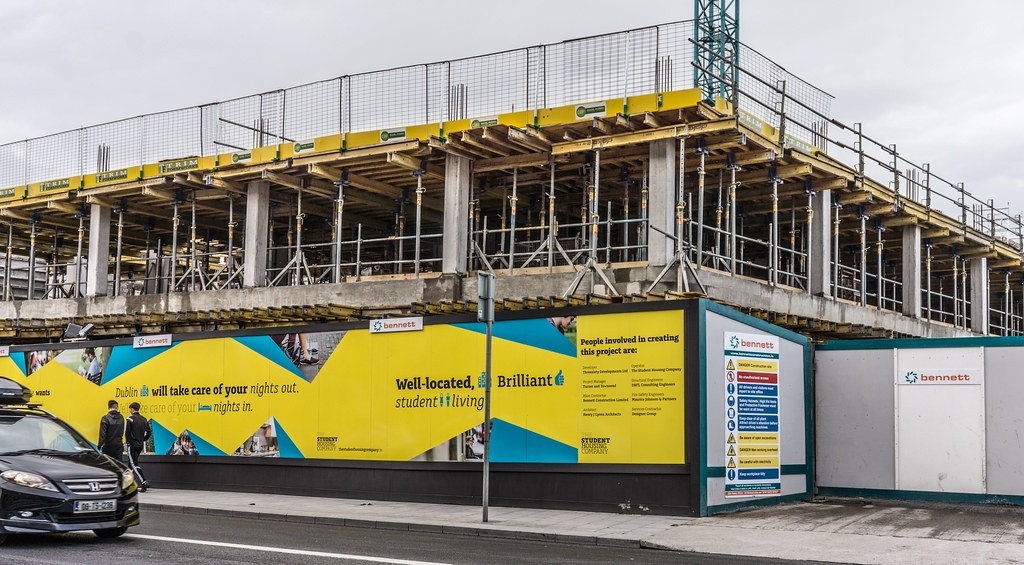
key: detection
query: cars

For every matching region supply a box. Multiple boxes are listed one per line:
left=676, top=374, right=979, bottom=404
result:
left=0, top=376, right=140, bottom=547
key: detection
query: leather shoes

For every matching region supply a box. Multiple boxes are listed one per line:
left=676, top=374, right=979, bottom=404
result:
left=139, top=481, right=150, bottom=492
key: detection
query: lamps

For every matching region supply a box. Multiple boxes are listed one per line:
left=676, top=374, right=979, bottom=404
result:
left=79, top=323, right=97, bottom=341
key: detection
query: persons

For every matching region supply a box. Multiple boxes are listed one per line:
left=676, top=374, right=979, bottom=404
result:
left=97, top=400, right=124, bottom=462
left=174, top=431, right=191, bottom=455
left=30, top=353, right=46, bottom=373
left=548, top=315, right=576, bottom=335
left=79, top=347, right=102, bottom=386
left=125, top=402, right=152, bottom=492
left=298, top=333, right=320, bottom=365
left=466, top=436, right=481, bottom=459
